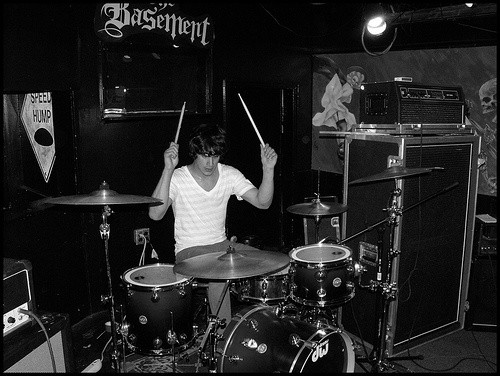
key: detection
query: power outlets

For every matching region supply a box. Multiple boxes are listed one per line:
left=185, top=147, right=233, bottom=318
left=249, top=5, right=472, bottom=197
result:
left=133, top=228, right=149, bottom=246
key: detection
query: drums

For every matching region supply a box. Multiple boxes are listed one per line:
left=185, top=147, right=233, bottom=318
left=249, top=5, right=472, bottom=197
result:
left=120, top=263, right=196, bottom=354
left=216, top=304, right=356, bottom=374
left=232, top=265, right=291, bottom=301
left=288, top=244, right=355, bottom=308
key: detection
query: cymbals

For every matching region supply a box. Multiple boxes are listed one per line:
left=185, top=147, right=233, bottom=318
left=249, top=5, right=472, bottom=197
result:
left=174, top=251, right=291, bottom=280
left=286, top=202, right=350, bottom=216
left=39, top=189, right=163, bottom=206
left=348, top=166, right=446, bottom=185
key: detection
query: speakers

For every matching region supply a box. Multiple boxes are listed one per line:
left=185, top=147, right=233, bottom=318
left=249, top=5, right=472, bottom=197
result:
left=345, top=132, right=483, bottom=359
left=4, top=329, right=67, bottom=374
left=466, top=259, right=497, bottom=332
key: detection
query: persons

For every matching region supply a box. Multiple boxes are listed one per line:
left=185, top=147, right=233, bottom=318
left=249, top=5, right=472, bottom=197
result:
left=148, top=140, right=277, bottom=335
